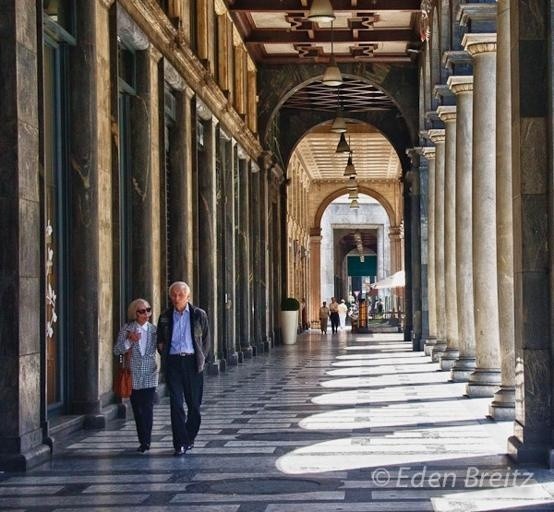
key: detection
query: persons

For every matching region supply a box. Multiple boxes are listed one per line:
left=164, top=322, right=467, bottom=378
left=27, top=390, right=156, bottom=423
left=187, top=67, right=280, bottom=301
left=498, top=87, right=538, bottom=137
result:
left=112, top=298, right=164, bottom=455
left=155, top=280, right=212, bottom=458
left=318, top=301, right=331, bottom=335
left=301, top=296, right=308, bottom=330
left=327, top=297, right=339, bottom=335
left=337, top=299, right=348, bottom=330
left=367, top=297, right=385, bottom=319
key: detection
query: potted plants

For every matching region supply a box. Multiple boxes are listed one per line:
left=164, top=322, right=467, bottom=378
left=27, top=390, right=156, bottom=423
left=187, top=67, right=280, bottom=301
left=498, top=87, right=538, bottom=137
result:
left=280, top=298, right=300, bottom=344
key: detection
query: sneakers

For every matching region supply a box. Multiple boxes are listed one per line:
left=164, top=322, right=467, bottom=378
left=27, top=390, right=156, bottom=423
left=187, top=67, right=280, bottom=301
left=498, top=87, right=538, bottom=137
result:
left=141, top=443, right=150, bottom=454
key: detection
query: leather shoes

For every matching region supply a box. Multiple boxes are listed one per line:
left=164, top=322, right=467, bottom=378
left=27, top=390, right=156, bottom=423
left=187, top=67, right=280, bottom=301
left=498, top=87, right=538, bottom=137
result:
left=176, top=437, right=194, bottom=456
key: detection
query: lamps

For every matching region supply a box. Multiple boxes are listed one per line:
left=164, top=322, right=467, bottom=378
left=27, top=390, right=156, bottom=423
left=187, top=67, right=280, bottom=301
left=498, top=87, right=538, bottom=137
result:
left=343, top=135, right=360, bottom=209
left=308, top=0, right=336, bottom=22
left=330, top=88, right=352, bottom=155
left=323, top=20, right=344, bottom=86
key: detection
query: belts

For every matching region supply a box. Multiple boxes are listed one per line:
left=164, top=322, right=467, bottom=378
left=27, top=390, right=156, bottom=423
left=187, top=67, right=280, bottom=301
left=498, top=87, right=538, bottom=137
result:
left=171, top=352, right=189, bottom=357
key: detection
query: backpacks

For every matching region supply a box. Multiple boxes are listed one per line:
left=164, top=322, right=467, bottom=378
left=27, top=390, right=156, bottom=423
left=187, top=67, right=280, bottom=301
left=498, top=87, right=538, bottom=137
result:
left=112, top=367, right=132, bottom=397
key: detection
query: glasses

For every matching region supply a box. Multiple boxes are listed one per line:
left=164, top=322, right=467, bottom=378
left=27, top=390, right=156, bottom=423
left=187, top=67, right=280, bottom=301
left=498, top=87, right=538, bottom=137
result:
left=136, top=308, right=151, bottom=314
